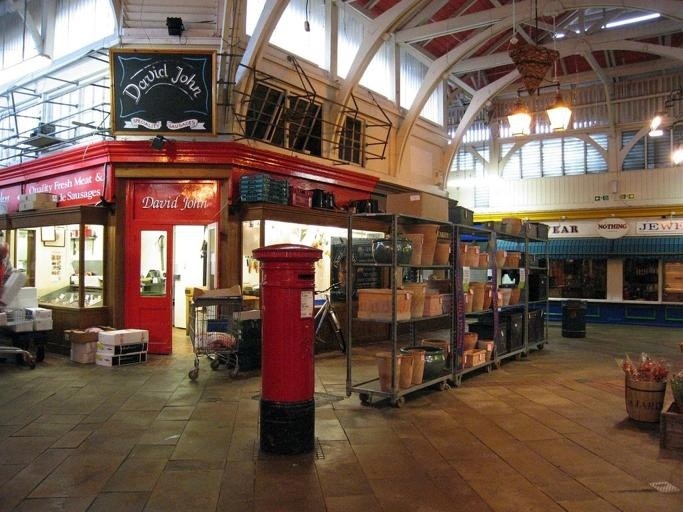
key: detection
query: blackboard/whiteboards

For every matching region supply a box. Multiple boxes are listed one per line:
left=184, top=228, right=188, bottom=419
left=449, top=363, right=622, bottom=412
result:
left=330, top=244, right=382, bottom=303
left=109, top=47, right=218, bottom=138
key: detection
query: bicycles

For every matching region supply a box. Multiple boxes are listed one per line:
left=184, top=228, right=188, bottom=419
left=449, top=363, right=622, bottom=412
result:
left=315, top=281, right=346, bottom=355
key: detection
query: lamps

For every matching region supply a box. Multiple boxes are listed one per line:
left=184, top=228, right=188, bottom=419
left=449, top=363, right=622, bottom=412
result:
left=505, top=0, right=682, bottom=167
left=166, top=16, right=182, bottom=35
left=149, top=136, right=170, bottom=150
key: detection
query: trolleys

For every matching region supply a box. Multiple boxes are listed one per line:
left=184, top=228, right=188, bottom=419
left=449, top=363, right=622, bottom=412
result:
left=0, top=307, right=36, bottom=369
left=186, top=294, right=243, bottom=379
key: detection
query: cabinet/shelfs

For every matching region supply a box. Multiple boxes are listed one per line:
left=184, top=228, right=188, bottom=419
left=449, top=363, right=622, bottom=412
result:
left=346, top=211, right=549, bottom=409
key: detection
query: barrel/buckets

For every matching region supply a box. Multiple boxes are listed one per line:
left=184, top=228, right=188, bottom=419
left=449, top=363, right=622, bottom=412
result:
left=375, top=223, right=520, bottom=393
left=624, top=376, right=666, bottom=422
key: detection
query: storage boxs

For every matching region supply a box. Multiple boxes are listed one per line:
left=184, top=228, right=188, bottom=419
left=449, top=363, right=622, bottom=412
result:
left=377, top=192, right=551, bottom=240
left=240, top=173, right=334, bottom=209
left=18, top=192, right=57, bottom=212
left=0, top=271, right=149, bottom=367
